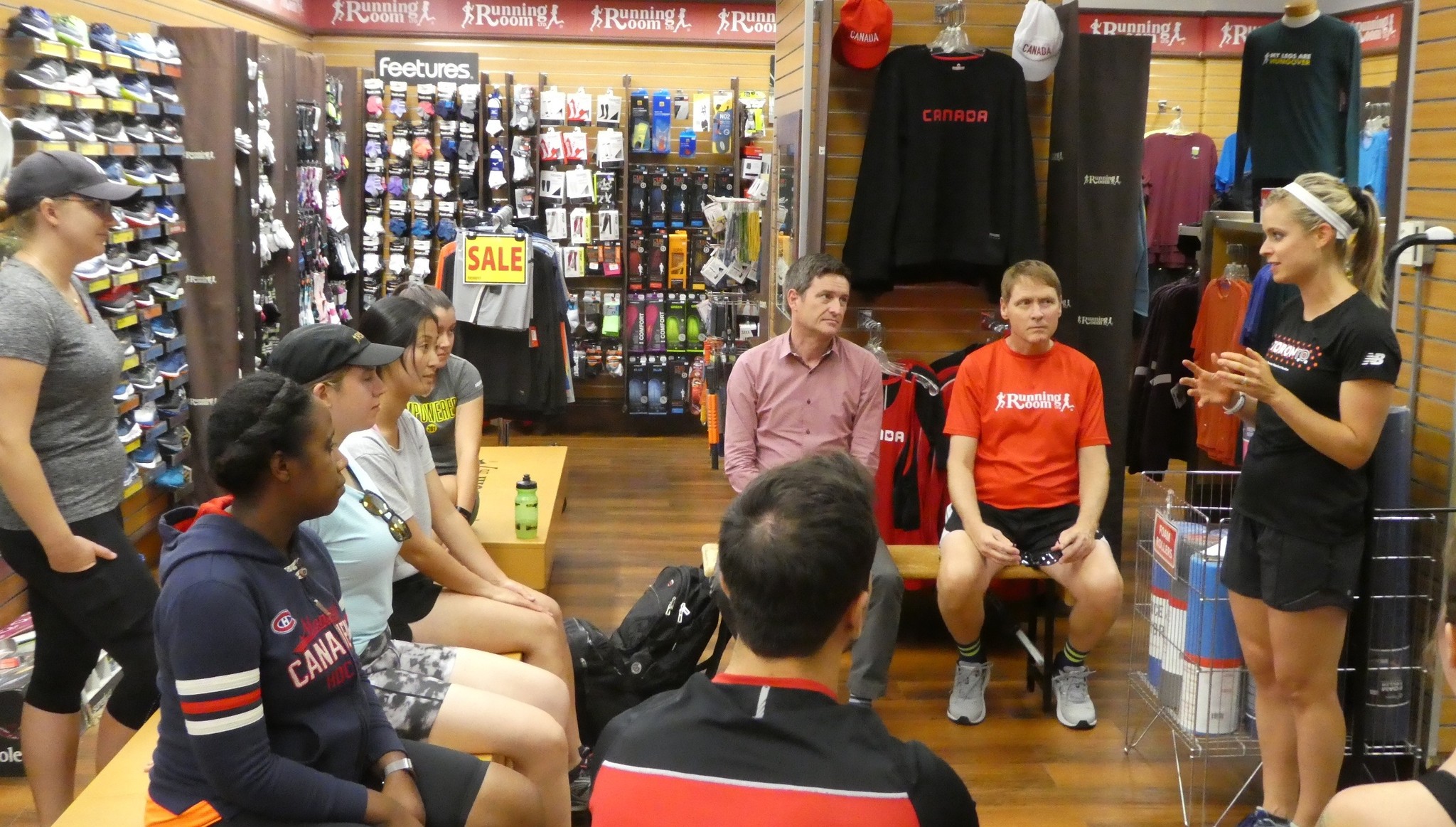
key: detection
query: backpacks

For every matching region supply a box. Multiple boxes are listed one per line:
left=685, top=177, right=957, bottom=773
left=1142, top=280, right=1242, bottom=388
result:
left=610, top=564, right=733, bottom=694
left=563, top=618, right=638, bottom=747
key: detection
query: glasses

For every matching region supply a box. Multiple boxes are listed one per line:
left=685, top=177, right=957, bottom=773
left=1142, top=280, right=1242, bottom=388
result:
left=360, top=488, right=412, bottom=542
left=1011, top=519, right=1063, bottom=567
left=32, top=192, right=110, bottom=216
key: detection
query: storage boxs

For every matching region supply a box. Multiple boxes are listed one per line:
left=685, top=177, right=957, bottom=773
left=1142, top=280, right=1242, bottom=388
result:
left=740, top=145, right=764, bottom=180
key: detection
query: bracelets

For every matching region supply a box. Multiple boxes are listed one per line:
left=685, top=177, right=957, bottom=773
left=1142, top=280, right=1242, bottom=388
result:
left=1222, top=391, right=1245, bottom=415
left=455, top=506, right=472, bottom=521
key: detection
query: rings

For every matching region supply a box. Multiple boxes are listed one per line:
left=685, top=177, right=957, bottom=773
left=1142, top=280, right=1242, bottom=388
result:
left=1243, top=377, right=1248, bottom=384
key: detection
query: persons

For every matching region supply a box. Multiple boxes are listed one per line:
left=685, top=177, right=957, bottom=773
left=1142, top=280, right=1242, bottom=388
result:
left=259, top=325, right=582, bottom=827
left=720, top=252, right=903, bottom=716
left=353, top=295, right=599, bottom=827
left=1176, top=170, right=1401, bottom=827
left=389, top=281, right=486, bottom=556
left=148, top=369, right=543, bottom=827
left=0, top=148, right=166, bottom=827
left=582, top=454, right=982, bottom=827
left=937, top=260, right=1127, bottom=734
left=1235, top=0, right=1361, bottom=187
left=1310, top=559, right=1456, bottom=827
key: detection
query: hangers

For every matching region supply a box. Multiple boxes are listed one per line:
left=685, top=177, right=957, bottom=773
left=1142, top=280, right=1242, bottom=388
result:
left=861, top=314, right=1011, bottom=396
left=1215, top=241, right=1253, bottom=293
left=1144, top=105, right=1194, bottom=140
left=1185, top=264, right=1202, bottom=292
left=923, top=0, right=986, bottom=58
left=474, top=203, right=559, bottom=259
left=1362, top=100, right=1393, bottom=135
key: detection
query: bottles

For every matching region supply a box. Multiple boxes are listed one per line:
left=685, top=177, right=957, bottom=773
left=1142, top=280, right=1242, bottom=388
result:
left=515, top=474, right=538, bottom=539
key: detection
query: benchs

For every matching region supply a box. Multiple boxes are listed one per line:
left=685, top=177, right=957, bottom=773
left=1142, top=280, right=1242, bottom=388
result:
left=45, top=442, right=575, bottom=827
left=700, top=541, right=1074, bottom=718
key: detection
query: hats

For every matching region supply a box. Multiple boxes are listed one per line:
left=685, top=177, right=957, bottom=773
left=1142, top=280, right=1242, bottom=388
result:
left=5, top=148, right=143, bottom=208
left=832, top=0, right=893, bottom=71
left=1011, top=0, right=1064, bottom=81
left=268, top=323, right=406, bottom=384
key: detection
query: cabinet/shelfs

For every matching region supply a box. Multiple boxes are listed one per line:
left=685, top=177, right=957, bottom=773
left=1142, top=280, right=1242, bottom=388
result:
left=0, top=16, right=359, bottom=500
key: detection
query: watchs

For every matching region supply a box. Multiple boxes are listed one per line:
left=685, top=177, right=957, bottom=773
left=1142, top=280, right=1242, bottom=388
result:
left=383, top=758, right=418, bottom=781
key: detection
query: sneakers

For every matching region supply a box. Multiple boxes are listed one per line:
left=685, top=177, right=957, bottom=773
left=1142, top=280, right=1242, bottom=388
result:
left=946, top=656, right=994, bottom=724
left=3, top=6, right=196, bottom=492
left=1235, top=807, right=1290, bottom=827
left=1050, top=652, right=1098, bottom=728
left=569, top=745, right=591, bottom=811
